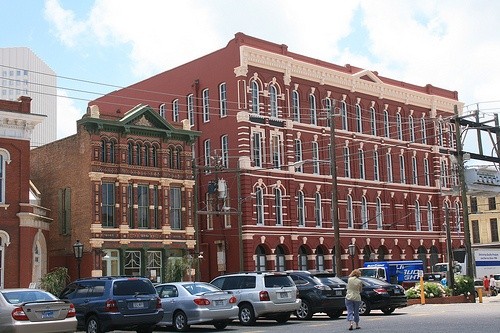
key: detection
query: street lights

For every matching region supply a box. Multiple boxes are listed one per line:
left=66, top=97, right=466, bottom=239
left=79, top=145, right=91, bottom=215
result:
left=72, top=239, right=84, bottom=281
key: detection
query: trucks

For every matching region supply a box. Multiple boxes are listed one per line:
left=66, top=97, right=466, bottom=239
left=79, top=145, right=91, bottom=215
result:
left=358, top=260, right=424, bottom=290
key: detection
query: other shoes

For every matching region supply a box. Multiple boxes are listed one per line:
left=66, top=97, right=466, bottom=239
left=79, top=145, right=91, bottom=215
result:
left=356, top=326, right=360, bottom=329
left=349, top=324, right=353, bottom=331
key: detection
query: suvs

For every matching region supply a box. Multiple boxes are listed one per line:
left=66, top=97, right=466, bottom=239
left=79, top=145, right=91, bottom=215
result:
left=209, top=271, right=301, bottom=325
left=58, top=275, right=164, bottom=333
left=286, top=270, right=349, bottom=321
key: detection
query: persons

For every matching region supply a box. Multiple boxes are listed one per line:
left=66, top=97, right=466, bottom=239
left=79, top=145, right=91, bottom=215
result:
left=489, top=274, right=497, bottom=296
left=483, top=275, right=490, bottom=297
left=344, top=269, right=362, bottom=330
left=440, top=275, right=447, bottom=287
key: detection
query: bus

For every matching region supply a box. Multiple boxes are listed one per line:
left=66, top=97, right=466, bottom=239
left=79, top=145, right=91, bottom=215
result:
left=454, top=243, right=500, bottom=281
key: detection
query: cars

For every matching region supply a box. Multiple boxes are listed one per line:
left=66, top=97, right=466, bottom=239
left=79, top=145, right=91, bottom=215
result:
left=154, top=282, right=239, bottom=333
left=0, top=288, right=78, bottom=333
left=493, top=274, right=500, bottom=290
left=474, top=279, right=486, bottom=297
left=434, top=263, right=448, bottom=278
left=340, top=277, right=409, bottom=316
